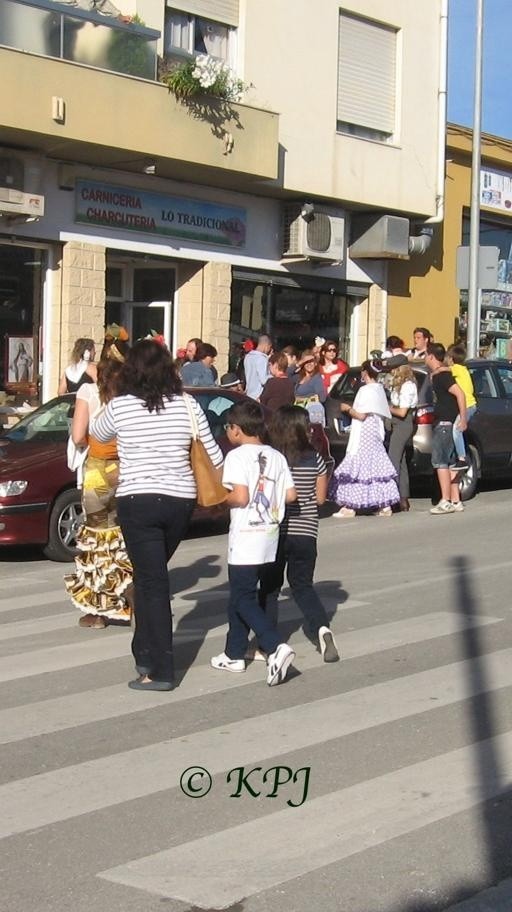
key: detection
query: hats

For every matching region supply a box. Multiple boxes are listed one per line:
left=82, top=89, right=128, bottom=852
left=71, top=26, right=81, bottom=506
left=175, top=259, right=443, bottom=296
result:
left=387, top=355, right=408, bottom=369
left=295, top=349, right=317, bottom=367
left=221, top=373, right=241, bottom=387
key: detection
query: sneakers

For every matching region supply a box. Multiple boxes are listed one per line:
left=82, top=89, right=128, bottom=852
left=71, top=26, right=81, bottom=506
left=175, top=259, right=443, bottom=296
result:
left=451, top=460, right=468, bottom=471
left=333, top=496, right=466, bottom=519
left=128, top=626, right=338, bottom=691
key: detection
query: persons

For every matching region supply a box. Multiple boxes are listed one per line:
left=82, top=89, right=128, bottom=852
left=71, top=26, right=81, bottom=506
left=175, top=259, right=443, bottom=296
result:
left=430, top=341, right=479, bottom=469
left=387, top=354, right=419, bottom=515
left=66, top=325, right=456, bottom=454
left=333, top=358, right=402, bottom=518
left=13, top=343, right=32, bottom=382
left=242, top=404, right=341, bottom=663
left=68, top=341, right=137, bottom=627
left=209, top=399, right=299, bottom=687
left=423, top=342, right=467, bottom=514
left=90, top=338, right=226, bottom=691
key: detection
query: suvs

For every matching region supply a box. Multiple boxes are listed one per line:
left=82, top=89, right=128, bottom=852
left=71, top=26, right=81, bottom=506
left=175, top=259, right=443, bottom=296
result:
left=323, top=357, right=511, bottom=501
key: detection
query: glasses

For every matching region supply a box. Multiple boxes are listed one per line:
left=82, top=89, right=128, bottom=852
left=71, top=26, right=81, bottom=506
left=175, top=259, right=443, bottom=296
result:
left=327, top=348, right=337, bottom=352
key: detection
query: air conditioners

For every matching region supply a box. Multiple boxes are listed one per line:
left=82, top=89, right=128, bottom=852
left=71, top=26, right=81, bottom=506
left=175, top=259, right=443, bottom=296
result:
left=281, top=200, right=346, bottom=265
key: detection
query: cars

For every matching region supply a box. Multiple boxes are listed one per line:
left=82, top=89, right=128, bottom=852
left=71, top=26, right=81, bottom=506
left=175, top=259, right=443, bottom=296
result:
left=0, top=387, right=336, bottom=569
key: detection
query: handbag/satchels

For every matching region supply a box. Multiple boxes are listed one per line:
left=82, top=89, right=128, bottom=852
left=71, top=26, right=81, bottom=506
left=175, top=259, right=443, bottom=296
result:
left=191, top=439, right=230, bottom=507
left=305, top=401, right=326, bottom=428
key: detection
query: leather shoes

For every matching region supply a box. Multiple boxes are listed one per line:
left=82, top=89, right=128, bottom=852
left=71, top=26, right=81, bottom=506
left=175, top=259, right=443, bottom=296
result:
left=78, top=614, right=106, bottom=628
